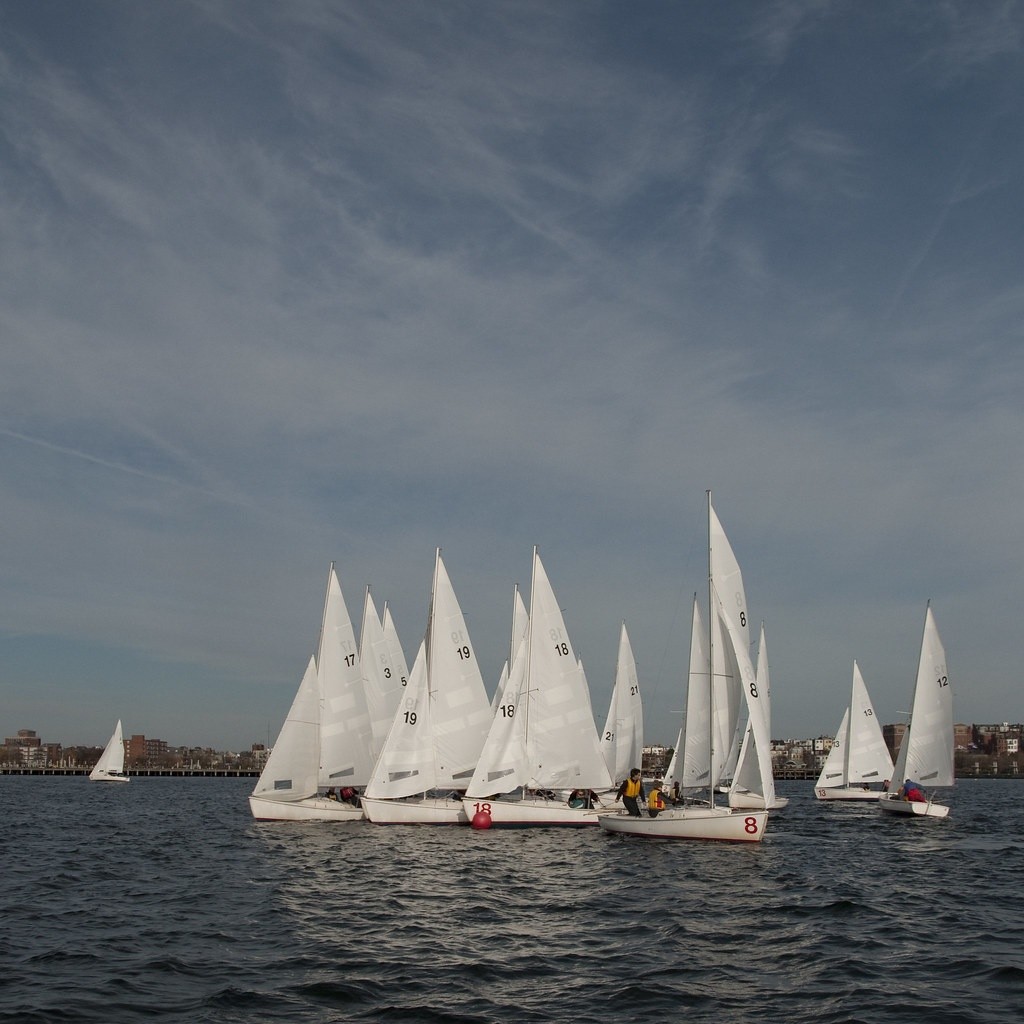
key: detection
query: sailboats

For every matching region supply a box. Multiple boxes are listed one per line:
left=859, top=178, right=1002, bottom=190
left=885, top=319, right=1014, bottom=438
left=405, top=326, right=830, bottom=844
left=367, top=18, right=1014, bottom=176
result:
left=878, top=597, right=954, bottom=818
left=89, top=719, right=130, bottom=782
left=248, top=489, right=789, bottom=842
left=813, top=660, right=896, bottom=799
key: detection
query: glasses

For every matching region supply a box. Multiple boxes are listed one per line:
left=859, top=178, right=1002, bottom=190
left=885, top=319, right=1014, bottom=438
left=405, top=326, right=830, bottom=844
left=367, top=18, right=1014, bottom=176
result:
left=578, top=793, right=582, bottom=796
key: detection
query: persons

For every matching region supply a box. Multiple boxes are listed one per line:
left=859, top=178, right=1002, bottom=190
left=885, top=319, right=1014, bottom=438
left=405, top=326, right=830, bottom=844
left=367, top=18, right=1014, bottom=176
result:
left=614, top=768, right=646, bottom=818
left=648, top=780, right=679, bottom=819
left=899, top=779, right=927, bottom=803
left=670, top=781, right=684, bottom=807
left=568, top=789, right=600, bottom=809
left=537, top=789, right=556, bottom=801
left=324, top=786, right=362, bottom=808
left=882, top=780, right=890, bottom=792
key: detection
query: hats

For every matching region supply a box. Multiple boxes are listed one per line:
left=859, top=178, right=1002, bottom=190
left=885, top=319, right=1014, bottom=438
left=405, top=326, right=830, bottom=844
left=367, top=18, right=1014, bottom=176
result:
left=653, top=780, right=663, bottom=788
